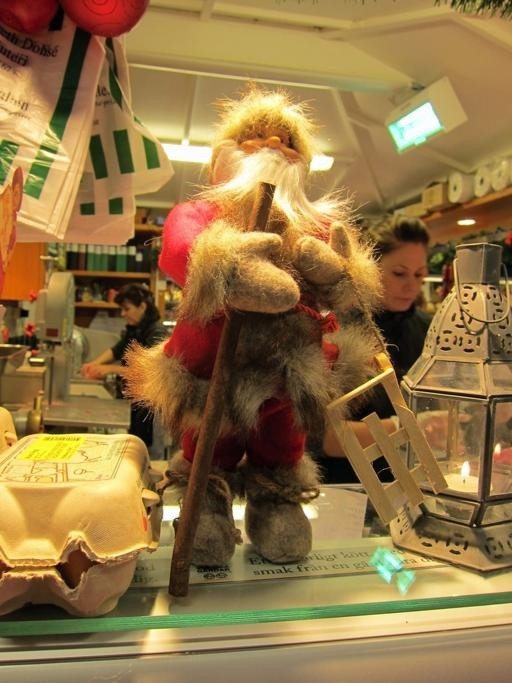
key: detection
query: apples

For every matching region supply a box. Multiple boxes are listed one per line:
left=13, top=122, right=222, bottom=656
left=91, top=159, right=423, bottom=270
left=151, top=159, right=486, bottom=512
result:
left=444, top=462, right=494, bottom=495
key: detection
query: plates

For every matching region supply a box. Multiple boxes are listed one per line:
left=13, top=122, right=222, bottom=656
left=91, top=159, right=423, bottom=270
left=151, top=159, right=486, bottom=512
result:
left=59, top=225, right=168, bottom=332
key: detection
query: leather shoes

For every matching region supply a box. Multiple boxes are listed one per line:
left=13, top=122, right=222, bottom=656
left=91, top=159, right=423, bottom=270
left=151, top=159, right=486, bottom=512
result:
left=293, top=222, right=382, bottom=320
left=179, top=222, right=299, bottom=321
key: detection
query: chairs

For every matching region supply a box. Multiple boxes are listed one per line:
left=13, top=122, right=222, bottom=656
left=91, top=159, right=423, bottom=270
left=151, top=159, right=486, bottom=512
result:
left=161, top=470, right=240, bottom=567
left=245, top=471, right=314, bottom=566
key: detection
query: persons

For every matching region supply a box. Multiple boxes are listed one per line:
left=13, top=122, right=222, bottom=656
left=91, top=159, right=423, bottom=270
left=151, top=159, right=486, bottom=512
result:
left=320, top=216, right=435, bottom=483
left=80, top=285, right=166, bottom=445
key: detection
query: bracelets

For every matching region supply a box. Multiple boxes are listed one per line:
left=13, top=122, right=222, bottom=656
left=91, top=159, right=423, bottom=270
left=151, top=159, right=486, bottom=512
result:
left=1, top=343, right=28, bottom=372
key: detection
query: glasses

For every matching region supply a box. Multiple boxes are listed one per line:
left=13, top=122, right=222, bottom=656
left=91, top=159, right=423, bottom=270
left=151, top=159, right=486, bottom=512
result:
left=386, top=76, right=468, bottom=154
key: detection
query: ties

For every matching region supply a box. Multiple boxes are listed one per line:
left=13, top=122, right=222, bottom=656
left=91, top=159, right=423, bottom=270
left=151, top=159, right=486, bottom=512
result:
left=385, top=76, right=470, bottom=158
